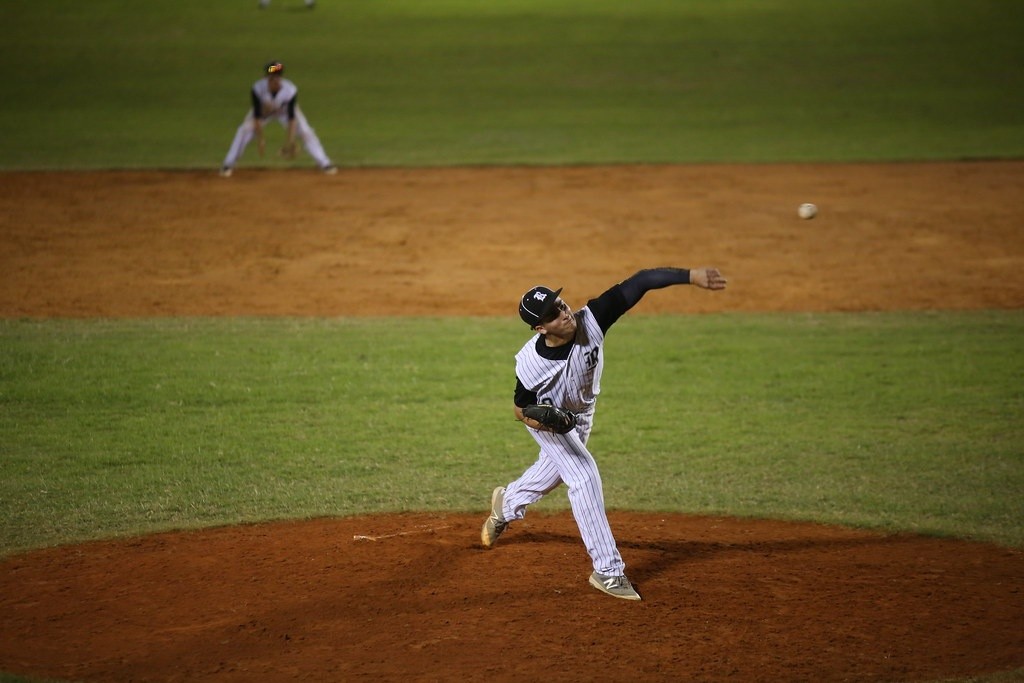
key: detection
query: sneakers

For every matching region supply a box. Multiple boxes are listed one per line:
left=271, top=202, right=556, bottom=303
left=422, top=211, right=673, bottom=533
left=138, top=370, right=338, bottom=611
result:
left=481, top=486, right=509, bottom=547
left=323, top=163, right=339, bottom=176
left=588, top=570, right=641, bottom=601
left=219, top=166, right=234, bottom=178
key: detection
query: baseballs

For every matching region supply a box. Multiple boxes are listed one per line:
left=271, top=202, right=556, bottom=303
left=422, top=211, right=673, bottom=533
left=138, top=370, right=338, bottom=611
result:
left=798, top=203, right=817, bottom=219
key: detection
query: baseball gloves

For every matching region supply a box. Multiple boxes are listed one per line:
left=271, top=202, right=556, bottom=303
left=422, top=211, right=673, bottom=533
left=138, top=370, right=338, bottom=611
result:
left=516, top=403, right=578, bottom=437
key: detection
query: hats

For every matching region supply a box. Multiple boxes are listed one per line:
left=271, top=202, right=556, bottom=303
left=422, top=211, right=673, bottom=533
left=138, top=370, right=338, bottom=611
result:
left=264, top=60, right=284, bottom=74
left=519, top=285, right=563, bottom=330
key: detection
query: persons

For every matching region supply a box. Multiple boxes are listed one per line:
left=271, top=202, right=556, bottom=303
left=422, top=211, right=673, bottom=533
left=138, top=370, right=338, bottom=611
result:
left=215, top=60, right=341, bottom=180
left=481, top=266, right=728, bottom=601
